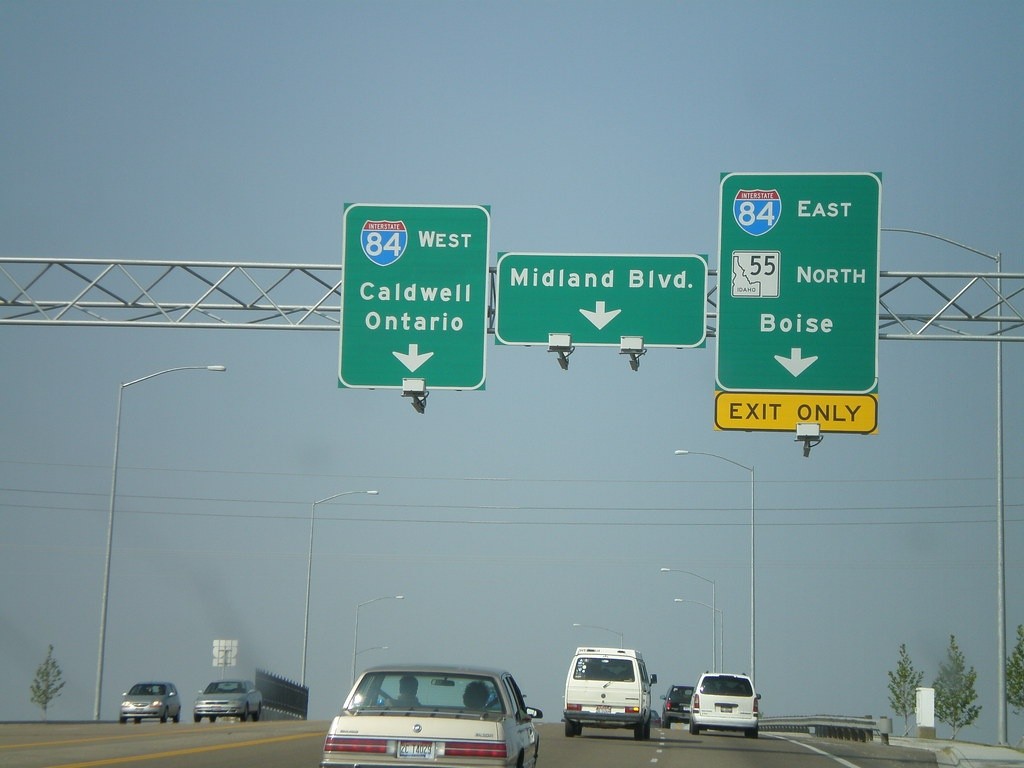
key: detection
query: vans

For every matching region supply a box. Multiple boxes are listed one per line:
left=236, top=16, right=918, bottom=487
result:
left=560, top=647, right=658, bottom=741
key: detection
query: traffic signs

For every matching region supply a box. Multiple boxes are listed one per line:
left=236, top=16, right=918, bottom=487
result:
left=337, top=203, right=491, bottom=392
left=712, top=171, right=883, bottom=393
left=494, top=251, right=708, bottom=350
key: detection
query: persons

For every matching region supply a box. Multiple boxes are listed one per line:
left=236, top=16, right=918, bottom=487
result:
left=383, top=676, right=420, bottom=707
left=463, top=681, right=490, bottom=707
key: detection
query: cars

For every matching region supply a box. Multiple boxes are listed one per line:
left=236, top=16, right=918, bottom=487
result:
left=650, top=709, right=661, bottom=728
left=119, top=682, right=181, bottom=723
left=319, top=664, right=543, bottom=768
left=193, top=680, right=263, bottom=722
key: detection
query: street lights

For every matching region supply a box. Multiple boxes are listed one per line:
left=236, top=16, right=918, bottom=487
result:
left=301, top=490, right=379, bottom=689
left=674, top=598, right=723, bottom=674
left=660, top=568, right=716, bottom=674
left=573, top=623, right=622, bottom=649
left=352, top=595, right=405, bottom=687
left=93, top=366, right=227, bottom=721
left=674, top=450, right=754, bottom=691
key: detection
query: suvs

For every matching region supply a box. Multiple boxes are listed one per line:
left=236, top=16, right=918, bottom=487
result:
left=689, top=670, right=761, bottom=738
left=659, top=685, right=696, bottom=729
